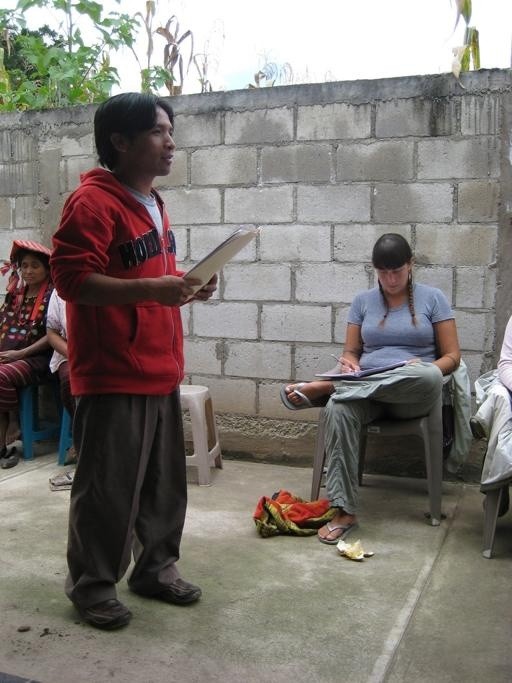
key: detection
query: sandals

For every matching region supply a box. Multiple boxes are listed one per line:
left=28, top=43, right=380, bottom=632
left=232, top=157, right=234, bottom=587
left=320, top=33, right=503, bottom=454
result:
left=0, top=446, right=19, bottom=469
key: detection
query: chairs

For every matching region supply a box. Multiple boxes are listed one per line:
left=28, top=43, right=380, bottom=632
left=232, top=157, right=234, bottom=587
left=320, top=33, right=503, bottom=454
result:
left=310, top=370, right=451, bottom=525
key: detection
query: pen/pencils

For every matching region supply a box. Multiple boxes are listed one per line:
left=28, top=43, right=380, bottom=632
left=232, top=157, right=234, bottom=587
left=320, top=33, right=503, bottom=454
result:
left=331, top=354, right=356, bottom=372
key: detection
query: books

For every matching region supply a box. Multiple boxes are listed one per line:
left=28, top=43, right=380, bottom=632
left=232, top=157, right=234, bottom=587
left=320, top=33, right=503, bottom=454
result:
left=313, top=360, right=409, bottom=381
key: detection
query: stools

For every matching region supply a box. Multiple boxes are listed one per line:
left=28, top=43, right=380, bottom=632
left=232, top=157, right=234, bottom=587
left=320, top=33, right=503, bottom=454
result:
left=18, top=380, right=65, bottom=458
left=56, top=408, right=77, bottom=466
left=481, top=482, right=512, bottom=558
left=180, top=385, right=222, bottom=487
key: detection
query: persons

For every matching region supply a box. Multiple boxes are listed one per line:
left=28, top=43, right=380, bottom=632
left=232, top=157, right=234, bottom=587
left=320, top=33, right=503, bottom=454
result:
left=48, top=92, right=215, bottom=632
left=0, top=239, right=83, bottom=468
left=280, top=233, right=461, bottom=545
left=496, top=315, right=512, bottom=393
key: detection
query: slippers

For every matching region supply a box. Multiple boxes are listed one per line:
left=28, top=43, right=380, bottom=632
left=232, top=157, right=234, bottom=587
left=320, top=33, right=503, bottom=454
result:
left=280, top=383, right=329, bottom=411
left=318, top=522, right=358, bottom=545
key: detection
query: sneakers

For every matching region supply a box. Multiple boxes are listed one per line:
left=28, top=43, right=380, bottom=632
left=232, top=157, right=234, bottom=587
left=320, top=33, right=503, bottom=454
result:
left=161, top=578, right=202, bottom=604
left=79, top=599, right=130, bottom=629
